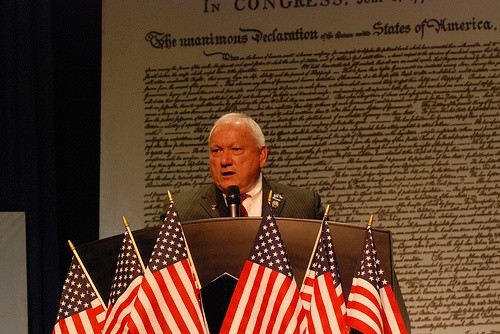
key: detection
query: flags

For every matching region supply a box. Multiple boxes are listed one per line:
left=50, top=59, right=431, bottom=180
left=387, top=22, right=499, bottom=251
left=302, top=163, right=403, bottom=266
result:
left=51, top=190, right=211, bottom=334
left=218, top=190, right=408, bottom=334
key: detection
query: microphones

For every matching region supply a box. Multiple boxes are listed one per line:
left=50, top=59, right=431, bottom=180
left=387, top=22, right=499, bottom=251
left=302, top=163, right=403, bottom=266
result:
left=227, top=185, right=240, bottom=217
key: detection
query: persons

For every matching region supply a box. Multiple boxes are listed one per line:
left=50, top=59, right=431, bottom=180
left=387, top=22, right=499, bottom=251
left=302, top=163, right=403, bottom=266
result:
left=159, top=112, right=329, bottom=221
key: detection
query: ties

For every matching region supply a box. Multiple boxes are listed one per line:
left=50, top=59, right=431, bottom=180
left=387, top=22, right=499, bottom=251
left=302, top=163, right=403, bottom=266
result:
left=240, top=193, right=252, bottom=217
